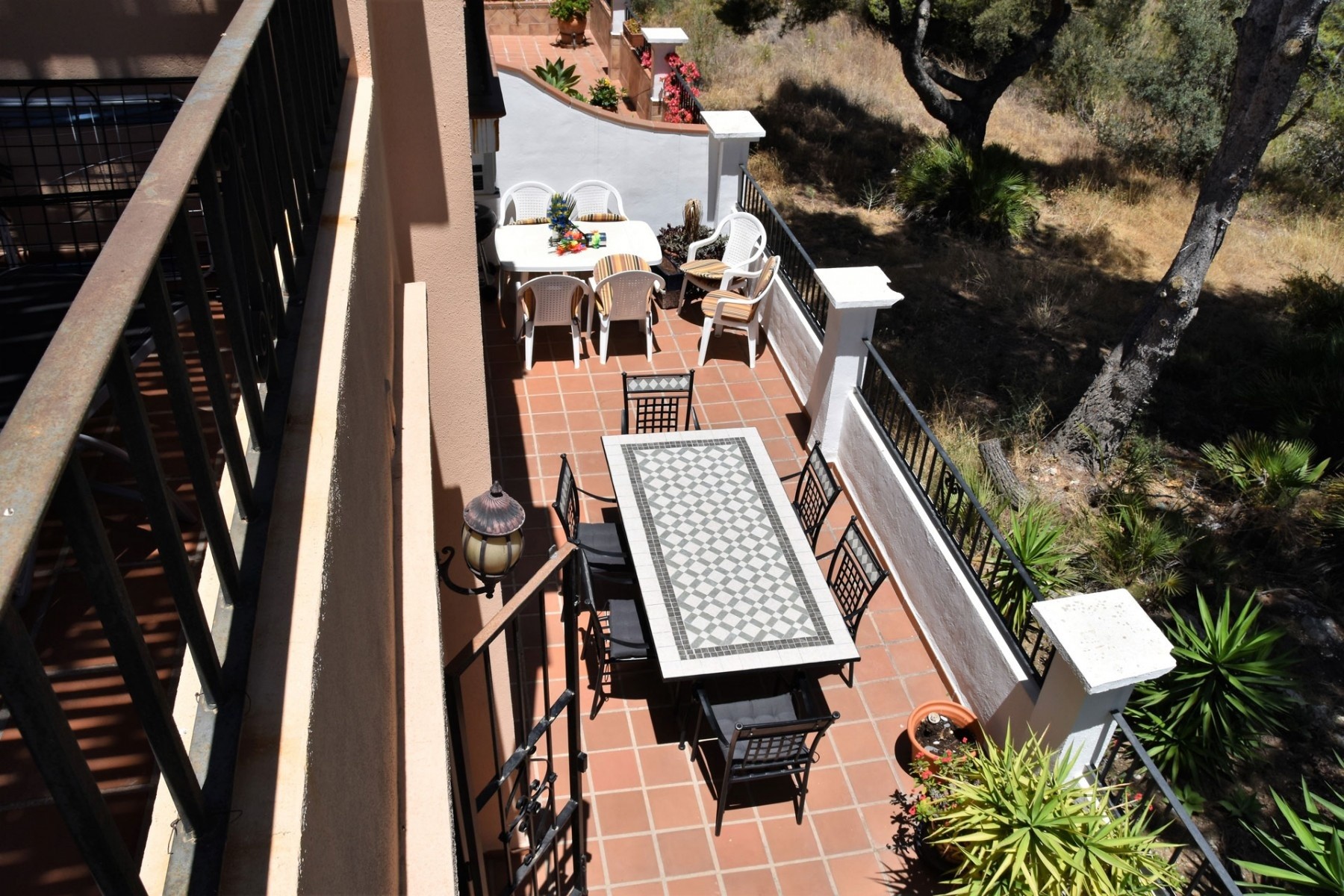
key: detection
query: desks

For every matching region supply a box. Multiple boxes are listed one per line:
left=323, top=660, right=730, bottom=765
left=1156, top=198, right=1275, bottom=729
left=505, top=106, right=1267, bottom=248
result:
left=603, top=423, right=862, bottom=750
left=493, top=221, right=662, bottom=336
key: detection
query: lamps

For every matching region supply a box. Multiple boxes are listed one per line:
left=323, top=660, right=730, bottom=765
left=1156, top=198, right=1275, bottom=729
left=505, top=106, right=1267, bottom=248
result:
left=475, top=201, right=498, bottom=247
left=436, top=480, right=528, bottom=600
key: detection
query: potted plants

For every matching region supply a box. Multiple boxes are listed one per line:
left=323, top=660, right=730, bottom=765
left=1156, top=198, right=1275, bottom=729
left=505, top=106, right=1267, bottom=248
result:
left=589, top=77, right=618, bottom=113
left=656, top=222, right=729, bottom=288
left=550, top=0, right=594, bottom=45
left=623, top=18, right=644, bottom=47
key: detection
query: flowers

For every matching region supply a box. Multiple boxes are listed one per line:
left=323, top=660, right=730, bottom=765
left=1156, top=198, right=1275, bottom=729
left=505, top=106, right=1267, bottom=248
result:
left=903, top=736, right=976, bottom=826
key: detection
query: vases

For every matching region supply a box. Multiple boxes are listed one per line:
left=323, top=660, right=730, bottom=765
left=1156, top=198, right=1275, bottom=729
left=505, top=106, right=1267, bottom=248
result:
left=905, top=700, right=984, bottom=861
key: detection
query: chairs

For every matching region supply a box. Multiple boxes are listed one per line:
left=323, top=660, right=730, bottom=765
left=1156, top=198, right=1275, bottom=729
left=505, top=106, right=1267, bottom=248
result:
left=691, top=677, right=840, bottom=837
left=497, top=177, right=665, bottom=369
left=620, top=371, right=702, bottom=435
left=551, top=452, right=655, bottom=719
left=779, top=440, right=890, bottom=688
left=678, top=212, right=780, bottom=368
